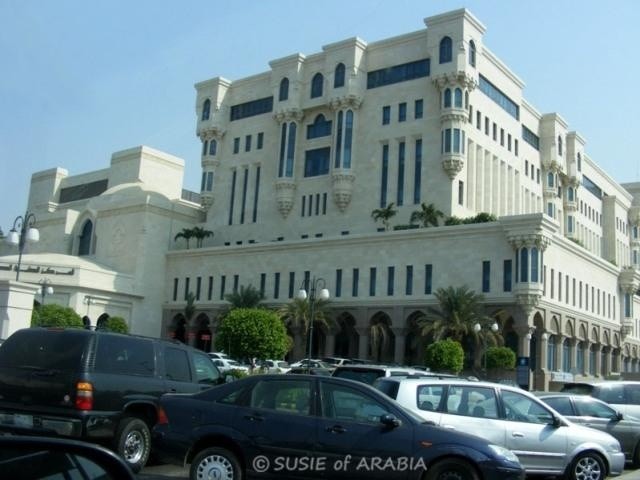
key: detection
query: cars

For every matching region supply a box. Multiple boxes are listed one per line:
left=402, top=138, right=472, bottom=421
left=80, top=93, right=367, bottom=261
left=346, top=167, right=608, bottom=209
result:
left=1, top=324, right=640, bottom=480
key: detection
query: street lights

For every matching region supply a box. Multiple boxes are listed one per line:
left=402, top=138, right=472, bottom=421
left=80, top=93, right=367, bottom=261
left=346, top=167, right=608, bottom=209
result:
left=5, top=210, right=40, bottom=281
left=473, top=318, right=500, bottom=382
left=296, top=274, right=331, bottom=375
left=37, top=276, right=54, bottom=307
